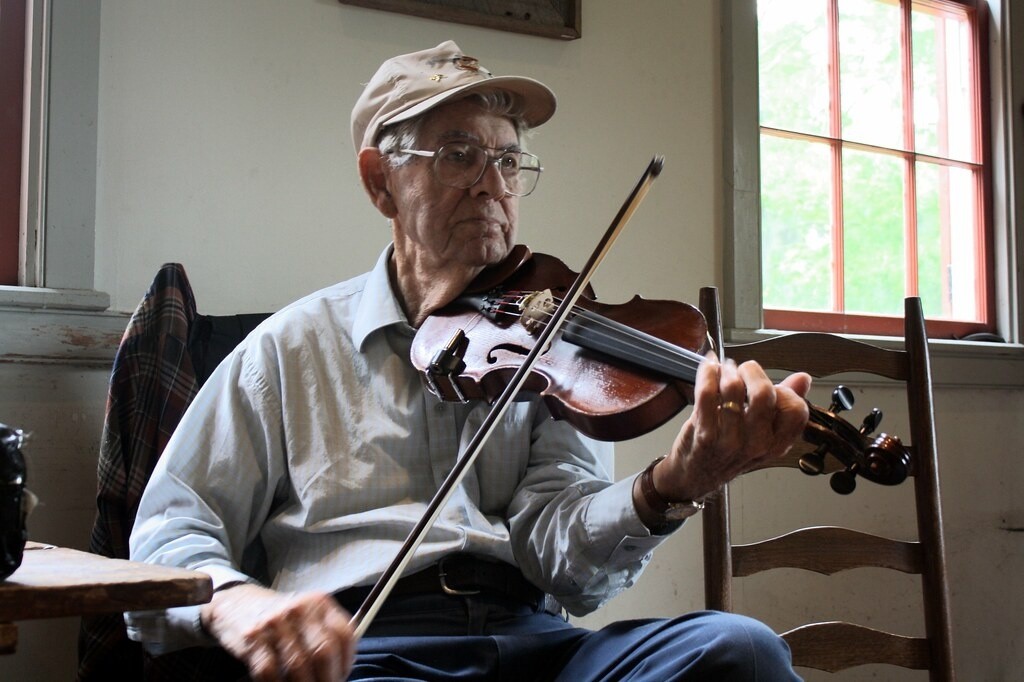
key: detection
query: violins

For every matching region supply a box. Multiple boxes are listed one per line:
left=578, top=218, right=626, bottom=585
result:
left=408, top=244, right=911, bottom=496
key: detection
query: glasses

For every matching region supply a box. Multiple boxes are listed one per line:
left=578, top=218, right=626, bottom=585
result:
left=386, top=142, right=544, bottom=197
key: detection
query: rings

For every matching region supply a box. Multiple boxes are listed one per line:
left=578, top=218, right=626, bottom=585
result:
left=719, top=402, right=743, bottom=413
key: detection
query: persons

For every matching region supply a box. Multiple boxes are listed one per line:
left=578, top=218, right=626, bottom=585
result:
left=120, top=42, right=814, bottom=682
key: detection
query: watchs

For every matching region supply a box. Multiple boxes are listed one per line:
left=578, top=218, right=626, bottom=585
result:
left=641, top=455, right=706, bottom=520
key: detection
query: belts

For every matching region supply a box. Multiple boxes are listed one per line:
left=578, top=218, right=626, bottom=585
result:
left=334, top=560, right=560, bottom=616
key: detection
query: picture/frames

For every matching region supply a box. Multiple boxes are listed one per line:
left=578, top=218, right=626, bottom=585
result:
left=337, top=0, right=582, bottom=41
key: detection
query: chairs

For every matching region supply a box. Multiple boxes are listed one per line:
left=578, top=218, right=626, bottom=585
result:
left=698, top=287, right=954, bottom=681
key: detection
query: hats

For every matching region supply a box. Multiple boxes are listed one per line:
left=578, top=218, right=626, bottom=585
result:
left=350, top=39, right=556, bottom=159
